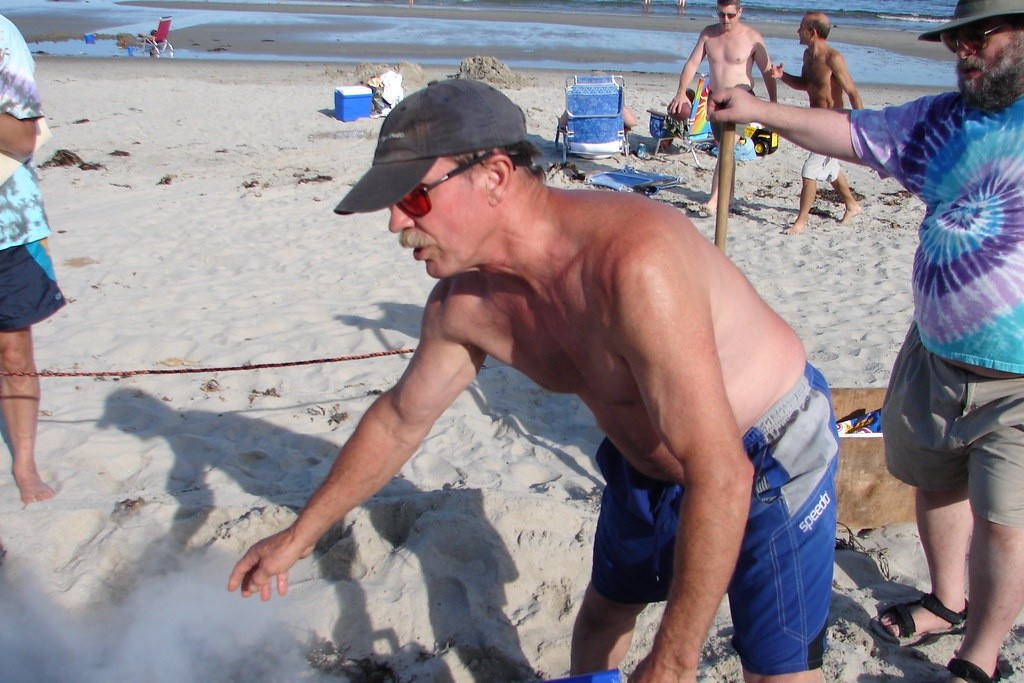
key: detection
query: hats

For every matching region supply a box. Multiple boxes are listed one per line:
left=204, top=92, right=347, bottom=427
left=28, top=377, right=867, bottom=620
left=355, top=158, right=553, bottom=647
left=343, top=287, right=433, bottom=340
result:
left=918, top=0, right=1024, bottom=42
left=333, top=79, right=527, bottom=215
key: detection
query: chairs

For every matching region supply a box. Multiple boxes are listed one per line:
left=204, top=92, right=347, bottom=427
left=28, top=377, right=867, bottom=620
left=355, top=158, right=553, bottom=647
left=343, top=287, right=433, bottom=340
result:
left=554, top=74, right=630, bottom=167
left=138, top=16, right=174, bottom=55
left=646, top=74, right=713, bottom=170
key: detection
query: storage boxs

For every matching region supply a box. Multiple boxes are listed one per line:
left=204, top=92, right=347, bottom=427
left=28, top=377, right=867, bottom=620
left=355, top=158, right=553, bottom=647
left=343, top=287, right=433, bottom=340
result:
left=334, top=85, right=374, bottom=122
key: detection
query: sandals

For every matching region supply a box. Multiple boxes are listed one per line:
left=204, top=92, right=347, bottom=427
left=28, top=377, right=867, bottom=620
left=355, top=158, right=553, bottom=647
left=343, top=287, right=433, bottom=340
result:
left=946, top=658, right=1001, bottom=683
left=871, top=594, right=968, bottom=647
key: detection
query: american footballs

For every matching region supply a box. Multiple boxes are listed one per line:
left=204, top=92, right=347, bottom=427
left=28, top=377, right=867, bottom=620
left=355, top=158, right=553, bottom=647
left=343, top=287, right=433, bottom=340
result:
left=666, top=102, right=692, bottom=122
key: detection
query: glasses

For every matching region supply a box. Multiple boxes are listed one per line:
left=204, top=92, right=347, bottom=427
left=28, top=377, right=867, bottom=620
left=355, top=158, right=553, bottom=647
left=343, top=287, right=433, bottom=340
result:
left=396, top=150, right=520, bottom=217
left=940, top=21, right=1011, bottom=53
left=716, top=8, right=740, bottom=19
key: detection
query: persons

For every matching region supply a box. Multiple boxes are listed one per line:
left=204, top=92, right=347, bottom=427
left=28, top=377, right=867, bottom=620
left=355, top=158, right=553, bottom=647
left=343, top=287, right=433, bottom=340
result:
left=226, top=78, right=840, bottom=683
left=706, top=0, right=1024, bottom=683
left=770, top=11, right=864, bottom=235
left=0, top=15, right=67, bottom=505
left=667, top=0, right=777, bottom=217
left=644, top=0, right=650, bottom=3
left=560, top=105, right=637, bottom=128
left=679, top=0, right=685, bottom=7
